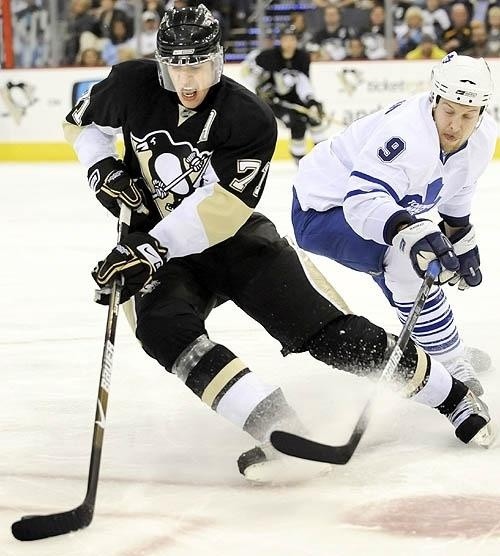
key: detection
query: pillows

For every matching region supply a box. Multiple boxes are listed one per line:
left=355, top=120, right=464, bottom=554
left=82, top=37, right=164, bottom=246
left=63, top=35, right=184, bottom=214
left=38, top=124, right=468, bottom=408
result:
left=430, top=51, right=493, bottom=128
left=279, top=26, right=297, bottom=34
left=154, top=4, right=224, bottom=92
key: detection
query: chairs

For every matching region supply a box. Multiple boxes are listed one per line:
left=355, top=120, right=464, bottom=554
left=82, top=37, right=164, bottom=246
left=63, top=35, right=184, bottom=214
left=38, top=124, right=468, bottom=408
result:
left=238, top=442, right=304, bottom=474
left=441, top=346, right=491, bottom=443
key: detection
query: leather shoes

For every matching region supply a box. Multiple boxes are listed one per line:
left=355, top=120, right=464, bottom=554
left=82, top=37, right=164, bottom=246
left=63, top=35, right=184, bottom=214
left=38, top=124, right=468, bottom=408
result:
left=91, top=231, right=168, bottom=305
left=88, top=156, right=149, bottom=223
left=392, top=219, right=482, bottom=291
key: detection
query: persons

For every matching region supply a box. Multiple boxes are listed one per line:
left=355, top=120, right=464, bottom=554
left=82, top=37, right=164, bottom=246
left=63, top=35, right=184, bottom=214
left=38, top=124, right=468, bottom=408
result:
left=243, top=30, right=331, bottom=166
left=291, top=51, right=497, bottom=397
left=61, top=2, right=491, bottom=476
left=52, top=1, right=191, bottom=69
left=288, top=0, right=500, bottom=59
